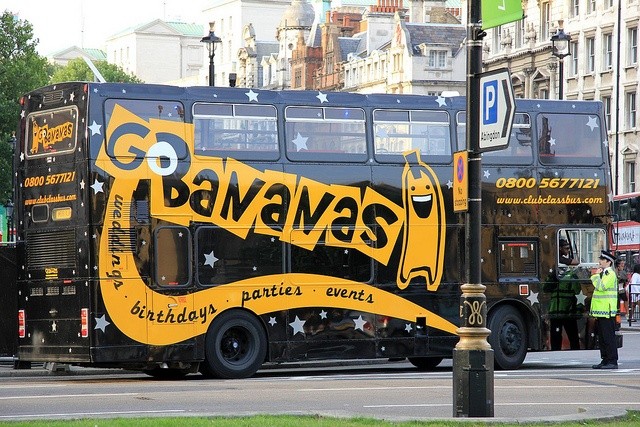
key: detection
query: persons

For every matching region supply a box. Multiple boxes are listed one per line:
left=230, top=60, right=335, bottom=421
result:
left=627, top=265, right=639, bottom=330
left=559, top=237, right=575, bottom=267
left=589, top=250, right=620, bottom=369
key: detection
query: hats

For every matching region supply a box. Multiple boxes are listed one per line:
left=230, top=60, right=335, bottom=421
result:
left=559, top=239, right=569, bottom=246
left=598, top=250, right=616, bottom=263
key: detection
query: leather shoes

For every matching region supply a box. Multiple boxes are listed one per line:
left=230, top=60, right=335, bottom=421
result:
left=600, top=362, right=618, bottom=369
left=592, top=363, right=604, bottom=369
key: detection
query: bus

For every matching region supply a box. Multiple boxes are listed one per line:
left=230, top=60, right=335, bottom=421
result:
left=11, top=79, right=620, bottom=376
left=611, top=192, right=640, bottom=315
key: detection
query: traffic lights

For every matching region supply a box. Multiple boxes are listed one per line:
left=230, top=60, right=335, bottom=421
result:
left=628, top=195, right=640, bottom=222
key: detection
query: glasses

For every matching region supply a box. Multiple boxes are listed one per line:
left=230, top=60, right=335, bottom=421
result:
left=562, top=247, right=570, bottom=250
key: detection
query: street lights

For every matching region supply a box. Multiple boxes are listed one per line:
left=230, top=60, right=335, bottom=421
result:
left=550, top=27, right=572, bottom=100
left=199, top=28, right=222, bottom=87
